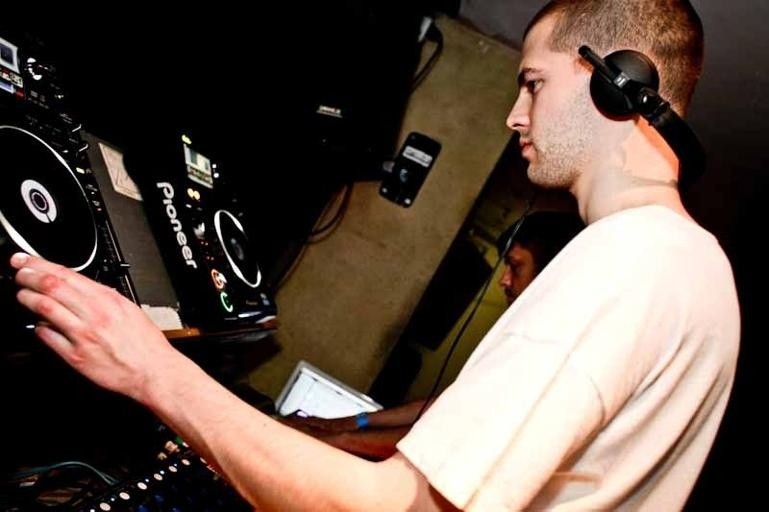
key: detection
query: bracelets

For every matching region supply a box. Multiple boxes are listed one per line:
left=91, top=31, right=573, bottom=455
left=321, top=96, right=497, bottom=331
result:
left=355, top=411, right=369, bottom=430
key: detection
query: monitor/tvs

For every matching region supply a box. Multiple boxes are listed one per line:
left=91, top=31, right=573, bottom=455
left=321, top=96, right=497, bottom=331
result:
left=276, top=361, right=385, bottom=419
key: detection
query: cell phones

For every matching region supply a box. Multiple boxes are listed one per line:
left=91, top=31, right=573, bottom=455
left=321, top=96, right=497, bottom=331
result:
left=379, top=131, right=441, bottom=208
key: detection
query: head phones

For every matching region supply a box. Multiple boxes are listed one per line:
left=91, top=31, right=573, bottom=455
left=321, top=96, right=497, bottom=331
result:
left=578, top=44, right=701, bottom=166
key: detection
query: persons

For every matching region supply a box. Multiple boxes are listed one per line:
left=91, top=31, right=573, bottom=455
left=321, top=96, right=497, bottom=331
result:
left=10, top=1, right=741, bottom=512
left=271, top=209, right=588, bottom=463
